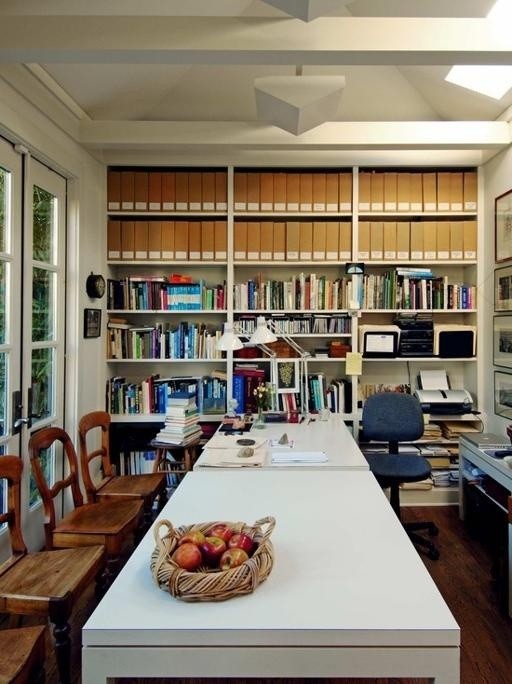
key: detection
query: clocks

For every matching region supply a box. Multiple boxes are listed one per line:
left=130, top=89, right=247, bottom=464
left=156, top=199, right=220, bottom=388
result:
left=87, top=271, right=106, bottom=299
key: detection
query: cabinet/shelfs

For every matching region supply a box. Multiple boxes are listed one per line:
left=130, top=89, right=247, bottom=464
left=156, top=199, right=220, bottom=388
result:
left=105, top=161, right=480, bottom=507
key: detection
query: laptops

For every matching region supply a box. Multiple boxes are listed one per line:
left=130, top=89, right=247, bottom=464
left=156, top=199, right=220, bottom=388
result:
left=460, top=431, right=512, bottom=449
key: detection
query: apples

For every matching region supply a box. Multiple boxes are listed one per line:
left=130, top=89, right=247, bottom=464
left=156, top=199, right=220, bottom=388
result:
left=228, top=534, right=253, bottom=553
left=200, top=536, right=227, bottom=560
left=220, top=548, right=249, bottom=572
left=177, top=529, right=205, bottom=548
left=172, top=543, right=202, bottom=570
left=208, top=524, right=233, bottom=542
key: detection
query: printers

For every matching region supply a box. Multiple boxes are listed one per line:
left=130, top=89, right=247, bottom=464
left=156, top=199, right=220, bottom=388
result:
left=411, top=374, right=474, bottom=416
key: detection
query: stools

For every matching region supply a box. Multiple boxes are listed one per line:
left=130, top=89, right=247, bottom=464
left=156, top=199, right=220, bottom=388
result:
left=149, top=437, right=200, bottom=475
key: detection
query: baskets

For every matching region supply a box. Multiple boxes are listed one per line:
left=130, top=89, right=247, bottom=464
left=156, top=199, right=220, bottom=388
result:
left=150, top=513, right=278, bottom=603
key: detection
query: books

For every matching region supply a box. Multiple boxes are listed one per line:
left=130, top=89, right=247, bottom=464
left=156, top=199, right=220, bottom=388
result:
left=279, top=394, right=302, bottom=414
left=312, top=319, right=350, bottom=333
left=302, top=370, right=351, bottom=414
left=165, top=452, right=181, bottom=487
left=232, top=271, right=352, bottom=310
left=107, top=369, right=226, bottom=416
left=270, top=319, right=288, bottom=334
left=233, top=319, right=256, bottom=335
left=151, top=391, right=204, bottom=447
left=118, top=450, right=157, bottom=474
left=232, top=363, right=264, bottom=414
left=290, top=320, right=310, bottom=333
left=107, top=317, right=224, bottom=359
left=358, top=268, right=476, bottom=309
left=107, top=274, right=228, bottom=310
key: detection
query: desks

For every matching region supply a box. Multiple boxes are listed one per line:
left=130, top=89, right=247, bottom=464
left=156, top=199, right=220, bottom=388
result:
left=192, top=408, right=371, bottom=470
left=79, top=470, right=463, bottom=684
left=458, top=433, right=512, bottom=620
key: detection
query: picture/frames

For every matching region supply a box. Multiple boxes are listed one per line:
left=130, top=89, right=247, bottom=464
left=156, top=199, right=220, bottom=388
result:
left=83, top=308, right=102, bottom=339
left=492, top=314, right=512, bottom=369
left=494, top=370, right=512, bottom=421
left=495, top=189, right=512, bottom=264
left=494, top=264, right=512, bottom=313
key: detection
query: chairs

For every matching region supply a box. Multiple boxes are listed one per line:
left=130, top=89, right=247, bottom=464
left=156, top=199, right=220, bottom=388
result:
left=0, top=454, right=110, bottom=683
left=28, top=427, right=145, bottom=572
left=361, top=391, right=441, bottom=561
left=78, top=411, right=169, bottom=523
